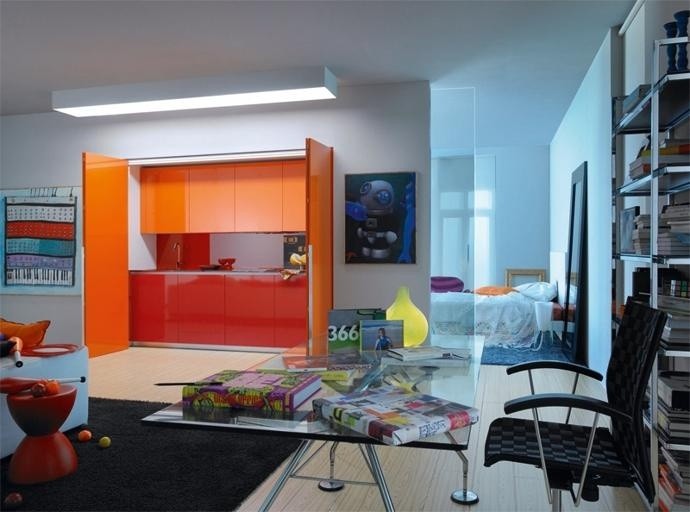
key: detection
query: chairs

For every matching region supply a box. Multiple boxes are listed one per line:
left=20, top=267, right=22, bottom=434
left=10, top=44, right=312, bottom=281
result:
left=482, top=296, right=668, bottom=510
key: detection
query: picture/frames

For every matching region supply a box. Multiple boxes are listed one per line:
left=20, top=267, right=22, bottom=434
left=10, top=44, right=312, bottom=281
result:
left=505, top=268, right=546, bottom=288
left=0, top=186, right=82, bottom=297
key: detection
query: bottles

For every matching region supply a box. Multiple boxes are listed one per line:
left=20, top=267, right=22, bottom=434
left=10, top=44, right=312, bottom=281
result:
left=386, top=285, right=427, bottom=348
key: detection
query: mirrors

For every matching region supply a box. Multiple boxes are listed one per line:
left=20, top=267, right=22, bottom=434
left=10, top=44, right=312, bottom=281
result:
left=559, top=161, right=589, bottom=363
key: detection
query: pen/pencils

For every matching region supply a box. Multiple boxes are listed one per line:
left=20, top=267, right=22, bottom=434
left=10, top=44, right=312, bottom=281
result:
left=287, top=368, right=327, bottom=373
left=155, top=382, right=222, bottom=386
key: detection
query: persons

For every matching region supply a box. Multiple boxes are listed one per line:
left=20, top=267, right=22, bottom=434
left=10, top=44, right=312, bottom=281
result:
left=374, top=328, right=394, bottom=351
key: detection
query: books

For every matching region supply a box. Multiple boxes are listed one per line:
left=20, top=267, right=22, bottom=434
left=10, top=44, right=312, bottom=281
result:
left=183, top=369, right=323, bottom=412
left=312, top=384, right=480, bottom=446
left=283, top=349, right=372, bottom=370
left=387, top=349, right=473, bottom=368
left=309, top=371, right=357, bottom=380
left=387, top=347, right=443, bottom=362
left=621, top=80, right=690, bottom=512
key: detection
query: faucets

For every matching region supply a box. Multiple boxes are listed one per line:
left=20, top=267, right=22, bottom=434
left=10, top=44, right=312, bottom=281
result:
left=173, top=241, right=181, bottom=263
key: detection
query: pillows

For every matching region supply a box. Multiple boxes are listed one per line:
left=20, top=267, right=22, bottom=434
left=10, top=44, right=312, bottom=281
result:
left=516, top=281, right=558, bottom=300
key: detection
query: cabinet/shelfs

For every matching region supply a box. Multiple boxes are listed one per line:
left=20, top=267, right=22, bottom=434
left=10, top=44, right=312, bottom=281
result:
left=609, top=36, right=689, bottom=512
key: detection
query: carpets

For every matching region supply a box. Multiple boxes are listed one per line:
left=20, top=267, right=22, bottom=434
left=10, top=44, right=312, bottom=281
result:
left=479, top=330, right=573, bottom=367
left=1, top=395, right=307, bottom=512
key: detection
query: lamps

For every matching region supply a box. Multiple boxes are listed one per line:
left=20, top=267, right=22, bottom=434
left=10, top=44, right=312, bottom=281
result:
left=52, top=69, right=336, bottom=119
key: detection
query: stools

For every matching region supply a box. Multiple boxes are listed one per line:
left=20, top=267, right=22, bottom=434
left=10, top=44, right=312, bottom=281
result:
left=5, top=382, right=81, bottom=484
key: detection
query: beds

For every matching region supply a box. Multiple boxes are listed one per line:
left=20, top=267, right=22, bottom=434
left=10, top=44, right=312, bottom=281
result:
left=429, top=281, right=561, bottom=337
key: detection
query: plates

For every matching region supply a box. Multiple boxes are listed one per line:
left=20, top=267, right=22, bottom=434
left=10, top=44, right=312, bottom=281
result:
left=200, top=264, right=221, bottom=271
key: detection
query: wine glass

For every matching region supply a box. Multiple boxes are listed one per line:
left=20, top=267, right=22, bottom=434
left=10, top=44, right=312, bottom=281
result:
left=218, top=258, right=236, bottom=271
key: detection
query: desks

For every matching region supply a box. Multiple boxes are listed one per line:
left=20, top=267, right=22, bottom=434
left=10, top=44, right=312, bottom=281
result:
left=142, top=328, right=480, bottom=512
